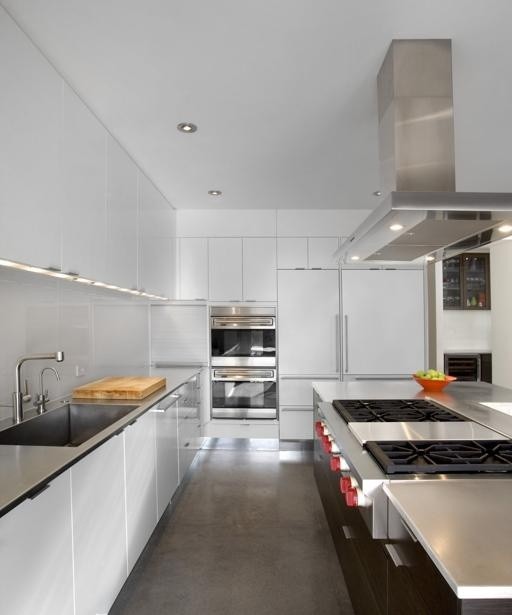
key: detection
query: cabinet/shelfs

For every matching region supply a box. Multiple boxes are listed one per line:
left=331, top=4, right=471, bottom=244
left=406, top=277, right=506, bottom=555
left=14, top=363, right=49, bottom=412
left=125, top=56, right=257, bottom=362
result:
left=176, top=228, right=352, bottom=304
left=109, top=136, right=176, bottom=306
left=151, top=386, right=182, bottom=526
left=124, top=396, right=156, bottom=579
left=1, top=467, right=75, bottom=615
left=276, top=266, right=428, bottom=444
left=3, top=11, right=106, bottom=287
left=176, top=371, right=203, bottom=487
left=68, top=429, right=128, bottom=615
left=305, top=391, right=510, bottom=615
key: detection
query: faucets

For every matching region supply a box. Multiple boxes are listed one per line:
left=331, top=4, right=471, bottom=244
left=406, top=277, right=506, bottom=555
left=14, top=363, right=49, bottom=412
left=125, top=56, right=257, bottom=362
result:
left=12, top=351, right=64, bottom=422
left=33, top=366, right=60, bottom=415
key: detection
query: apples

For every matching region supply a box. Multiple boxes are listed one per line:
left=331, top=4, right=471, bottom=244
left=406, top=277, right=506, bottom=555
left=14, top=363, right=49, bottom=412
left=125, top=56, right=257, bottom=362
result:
left=416, top=369, right=445, bottom=381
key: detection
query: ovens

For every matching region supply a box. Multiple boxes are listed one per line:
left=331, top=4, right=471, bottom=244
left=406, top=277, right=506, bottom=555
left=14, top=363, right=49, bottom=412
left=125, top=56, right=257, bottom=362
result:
left=210, top=316, right=277, bottom=418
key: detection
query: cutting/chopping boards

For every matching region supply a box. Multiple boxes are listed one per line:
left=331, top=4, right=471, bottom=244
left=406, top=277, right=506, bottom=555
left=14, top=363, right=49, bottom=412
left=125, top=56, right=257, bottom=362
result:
left=70, top=375, right=166, bottom=401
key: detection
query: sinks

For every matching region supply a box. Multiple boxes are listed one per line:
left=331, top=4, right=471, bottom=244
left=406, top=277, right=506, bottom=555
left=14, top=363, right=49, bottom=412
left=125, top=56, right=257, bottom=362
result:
left=0, top=403, right=140, bottom=448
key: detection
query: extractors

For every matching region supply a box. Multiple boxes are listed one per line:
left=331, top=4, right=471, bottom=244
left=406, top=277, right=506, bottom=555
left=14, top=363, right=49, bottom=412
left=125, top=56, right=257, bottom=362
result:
left=331, top=39, right=512, bottom=265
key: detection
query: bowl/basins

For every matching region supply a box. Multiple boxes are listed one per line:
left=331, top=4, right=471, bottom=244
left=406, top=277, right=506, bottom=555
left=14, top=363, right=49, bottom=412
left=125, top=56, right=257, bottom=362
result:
left=412, top=373, right=457, bottom=392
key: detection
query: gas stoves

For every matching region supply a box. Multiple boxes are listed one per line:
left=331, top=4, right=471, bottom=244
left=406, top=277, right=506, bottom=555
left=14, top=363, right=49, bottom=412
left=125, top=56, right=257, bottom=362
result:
left=315, top=394, right=511, bottom=540
